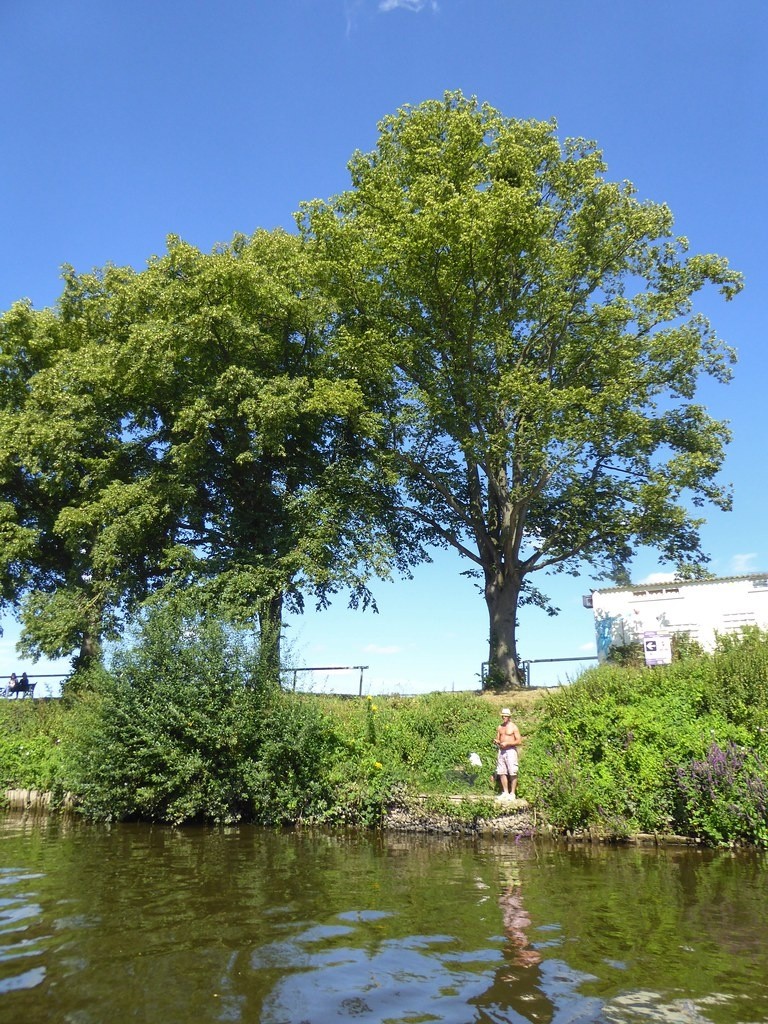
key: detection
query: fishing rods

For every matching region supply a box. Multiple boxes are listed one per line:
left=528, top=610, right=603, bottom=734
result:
left=448, top=709, right=502, bottom=746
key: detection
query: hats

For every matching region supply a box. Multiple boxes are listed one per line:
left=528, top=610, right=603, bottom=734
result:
left=500, top=708, right=512, bottom=716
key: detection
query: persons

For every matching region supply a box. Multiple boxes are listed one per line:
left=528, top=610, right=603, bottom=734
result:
left=7, top=672, right=29, bottom=700
left=0, top=672, right=18, bottom=698
left=492, top=709, right=522, bottom=801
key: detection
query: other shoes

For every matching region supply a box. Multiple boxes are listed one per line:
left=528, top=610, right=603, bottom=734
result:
left=506, top=793, right=515, bottom=800
left=497, top=792, right=509, bottom=799
